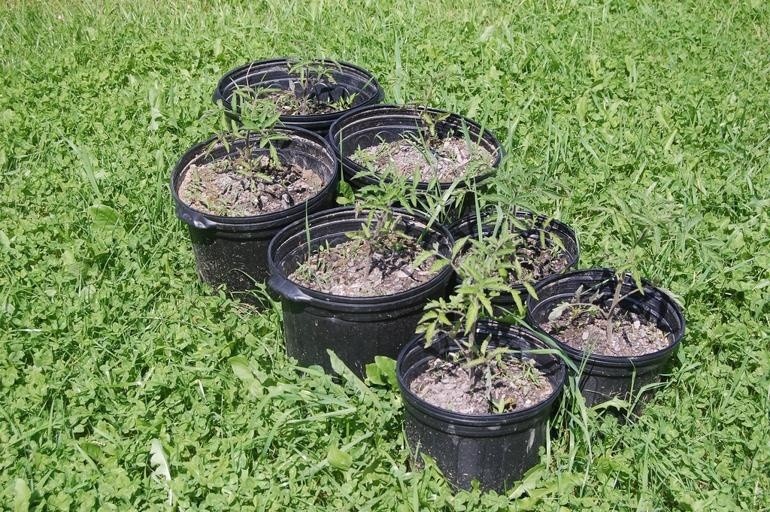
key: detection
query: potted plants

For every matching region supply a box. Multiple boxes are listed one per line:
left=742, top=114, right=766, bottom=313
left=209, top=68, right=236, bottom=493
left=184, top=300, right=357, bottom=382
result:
left=392, top=228, right=584, bottom=505
left=452, top=158, right=584, bottom=324
left=264, top=130, right=452, bottom=377
left=529, top=192, right=687, bottom=427
left=323, top=99, right=506, bottom=224
left=215, top=40, right=374, bottom=146
left=166, top=59, right=341, bottom=320
